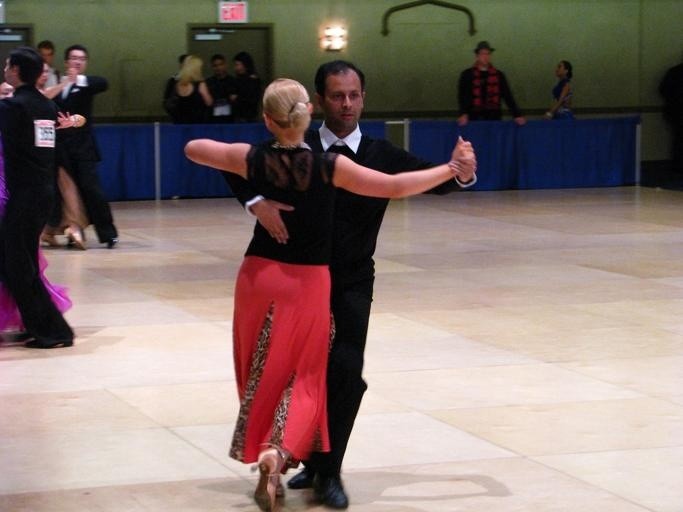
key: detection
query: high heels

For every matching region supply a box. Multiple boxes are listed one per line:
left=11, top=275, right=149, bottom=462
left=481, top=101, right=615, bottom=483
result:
left=251, top=449, right=283, bottom=512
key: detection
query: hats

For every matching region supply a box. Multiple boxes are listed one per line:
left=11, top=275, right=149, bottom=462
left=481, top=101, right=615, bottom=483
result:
left=474, top=41, right=494, bottom=53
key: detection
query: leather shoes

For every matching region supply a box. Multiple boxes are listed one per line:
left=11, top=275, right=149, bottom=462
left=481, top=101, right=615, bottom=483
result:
left=288, top=468, right=347, bottom=508
left=26, top=335, right=72, bottom=348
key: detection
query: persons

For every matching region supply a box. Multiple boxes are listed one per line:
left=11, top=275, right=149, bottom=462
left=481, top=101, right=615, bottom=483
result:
left=456, top=42, right=527, bottom=128
left=542, top=59, right=573, bottom=121
left=184, top=78, right=476, bottom=511
left=1, top=41, right=118, bottom=348
left=161, top=51, right=265, bottom=125
left=220, top=59, right=479, bottom=507
left=660, top=62, right=682, bottom=189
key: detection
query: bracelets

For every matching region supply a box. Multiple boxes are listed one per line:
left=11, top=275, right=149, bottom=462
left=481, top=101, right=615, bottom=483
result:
left=545, top=112, right=554, bottom=117
